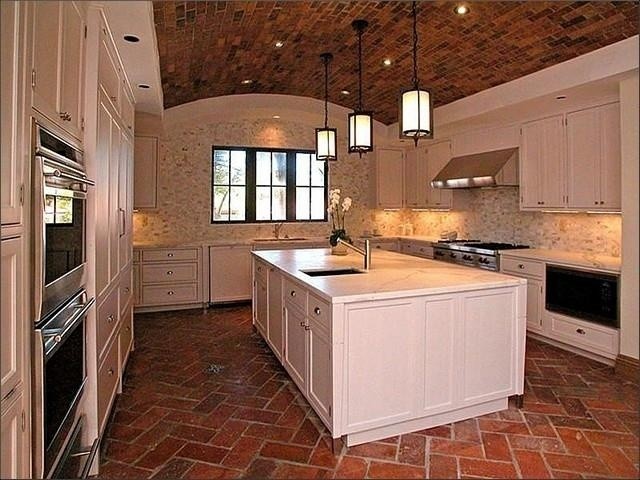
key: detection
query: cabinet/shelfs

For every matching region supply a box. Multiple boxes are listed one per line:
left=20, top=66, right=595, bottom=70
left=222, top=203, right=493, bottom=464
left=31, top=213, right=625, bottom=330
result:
left=404, top=142, right=428, bottom=208
left=134, top=132, right=160, bottom=215
left=566, top=101, right=623, bottom=211
left=428, top=138, right=453, bottom=210
left=141, top=246, right=202, bottom=313
left=518, top=113, right=565, bottom=211
left=282, top=277, right=345, bottom=439
left=367, top=145, right=405, bottom=209
left=209, top=246, right=252, bottom=303
left=253, top=259, right=283, bottom=367
left=359, top=242, right=619, bottom=368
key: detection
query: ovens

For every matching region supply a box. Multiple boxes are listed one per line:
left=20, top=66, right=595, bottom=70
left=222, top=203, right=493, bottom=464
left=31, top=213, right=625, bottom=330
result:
left=34, top=118, right=100, bottom=478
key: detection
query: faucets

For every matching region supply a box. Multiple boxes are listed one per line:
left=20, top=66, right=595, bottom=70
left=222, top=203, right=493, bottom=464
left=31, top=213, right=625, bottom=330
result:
left=336, top=237, right=371, bottom=270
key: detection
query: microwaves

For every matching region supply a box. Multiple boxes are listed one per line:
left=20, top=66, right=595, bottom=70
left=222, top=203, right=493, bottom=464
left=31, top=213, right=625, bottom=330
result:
left=544, top=263, right=620, bottom=329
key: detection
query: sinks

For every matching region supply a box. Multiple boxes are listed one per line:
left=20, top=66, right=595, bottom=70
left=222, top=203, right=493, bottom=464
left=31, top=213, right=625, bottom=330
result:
left=298, top=267, right=368, bottom=277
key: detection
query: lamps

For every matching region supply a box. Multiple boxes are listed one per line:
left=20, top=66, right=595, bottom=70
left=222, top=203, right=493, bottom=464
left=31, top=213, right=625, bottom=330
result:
left=348, top=19, right=374, bottom=158
left=398, top=0, right=434, bottom=146
left=315, top=53, right=337, bottom=161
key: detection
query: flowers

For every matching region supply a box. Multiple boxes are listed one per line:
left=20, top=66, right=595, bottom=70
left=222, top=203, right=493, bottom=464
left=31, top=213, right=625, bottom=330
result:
left=327, top=189, right=352, bottom=229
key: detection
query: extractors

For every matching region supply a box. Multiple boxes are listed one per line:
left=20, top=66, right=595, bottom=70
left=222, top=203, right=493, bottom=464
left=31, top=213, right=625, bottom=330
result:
left=429, top=148, right=521, bottom=192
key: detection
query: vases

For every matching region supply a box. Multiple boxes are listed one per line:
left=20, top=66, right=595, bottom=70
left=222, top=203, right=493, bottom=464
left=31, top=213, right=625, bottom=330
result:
left=329, top=229, right=352, bottom=254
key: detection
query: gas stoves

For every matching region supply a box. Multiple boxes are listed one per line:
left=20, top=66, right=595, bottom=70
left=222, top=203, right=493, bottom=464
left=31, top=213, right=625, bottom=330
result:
left=431, top=239, right=532, bottom=272
